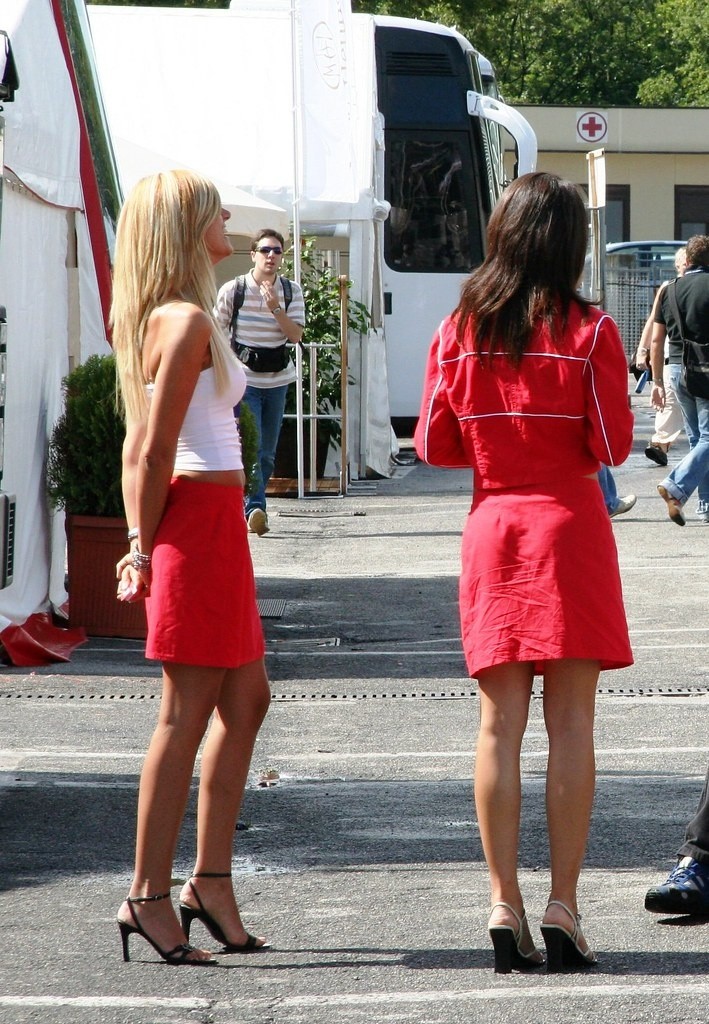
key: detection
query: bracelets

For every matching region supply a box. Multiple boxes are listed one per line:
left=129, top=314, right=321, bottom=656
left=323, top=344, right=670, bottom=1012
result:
left=128, top=528, right=138, bottom=541
left=132, top=543, right=151, bottom=570
left=638, top=348, right=647, bottom=352
left=653, top=378, right=663, bottom=382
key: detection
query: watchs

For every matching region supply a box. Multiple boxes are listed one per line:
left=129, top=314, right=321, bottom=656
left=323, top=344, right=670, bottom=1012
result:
left=271, top=304, right=281, bottom=314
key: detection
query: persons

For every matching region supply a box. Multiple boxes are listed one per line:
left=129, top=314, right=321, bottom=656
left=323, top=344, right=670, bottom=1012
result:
left=637, top=247, right=687, bottom=467
left=415, top=170, right=634, bottom=973
left=598, top=464, right=637, bottom=517
left=214, top=229, right=306, bottom=536
left=645, top=766, right=709, bottom=914
left=651, top=235, right=709, bottom=526
left=112, top=169, right=272, bottom=963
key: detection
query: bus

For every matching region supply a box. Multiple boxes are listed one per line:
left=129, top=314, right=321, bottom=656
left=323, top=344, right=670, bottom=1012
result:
left=76, top=0, right=540, bottom=451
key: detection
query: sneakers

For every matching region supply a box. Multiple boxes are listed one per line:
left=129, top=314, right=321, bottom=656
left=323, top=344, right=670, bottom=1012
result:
left=658, top=485, right=686, bottom=526
left=645, top=856, right=709, bottom=914
left=609, top=495, right=636, bottom=518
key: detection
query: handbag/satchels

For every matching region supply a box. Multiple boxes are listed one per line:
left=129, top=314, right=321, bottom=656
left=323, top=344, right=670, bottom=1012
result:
left=629, top=348, right=652, bottom=382
left=681, top=339, right=709, bottom=399
left=234, top=342, right=290, bottom=372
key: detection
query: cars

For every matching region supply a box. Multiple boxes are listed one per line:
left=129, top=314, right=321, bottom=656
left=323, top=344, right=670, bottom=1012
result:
left=576, top=239, right=689, bottom=300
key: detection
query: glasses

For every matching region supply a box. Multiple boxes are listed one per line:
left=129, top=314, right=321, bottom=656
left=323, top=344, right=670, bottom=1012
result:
left=254, top=246, right=282, bottom=254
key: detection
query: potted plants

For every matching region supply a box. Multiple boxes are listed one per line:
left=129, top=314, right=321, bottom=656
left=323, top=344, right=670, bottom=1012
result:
left=42, top=360, right=261, bottom=642
left=270, top=226, right=376, bottom=477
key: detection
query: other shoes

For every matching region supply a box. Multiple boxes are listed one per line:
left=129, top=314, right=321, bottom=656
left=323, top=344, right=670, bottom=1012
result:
left=248, top=508, right=270, bottom=536
left=645, top=443, right=667, bottom=466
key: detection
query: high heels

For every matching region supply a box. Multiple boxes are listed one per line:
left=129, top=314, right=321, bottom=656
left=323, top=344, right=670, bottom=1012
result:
left=540, top=901, right=598, bottom=971
left=117, top=892, right=216, bottom=965
left=179, top=873, right=270, bottom=950
left=488, top=902, right=546, bottom=974
